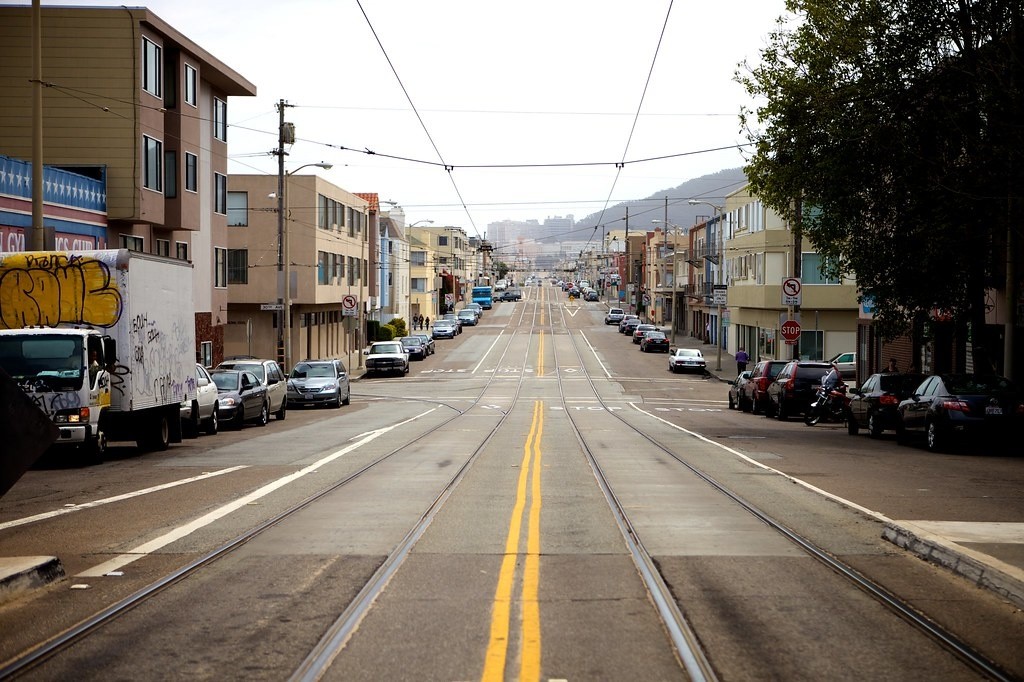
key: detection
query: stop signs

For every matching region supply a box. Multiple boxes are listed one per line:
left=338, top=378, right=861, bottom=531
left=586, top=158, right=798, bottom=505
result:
left=780, top=321, right=801, bottom=341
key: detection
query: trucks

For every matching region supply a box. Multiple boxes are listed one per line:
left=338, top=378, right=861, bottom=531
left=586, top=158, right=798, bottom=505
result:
left=0, top=250, right=198, bottom=466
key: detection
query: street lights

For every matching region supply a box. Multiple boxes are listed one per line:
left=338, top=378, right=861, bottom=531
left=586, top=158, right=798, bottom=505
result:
left=408, top=220, right=435, bottom=335
left=651, top=220, right=677, bottom=346
left=688, top=200, right=723, bottom=370
left=357, top=200, right=398, bottom=369
left=284, top=163, right=333, bottom=378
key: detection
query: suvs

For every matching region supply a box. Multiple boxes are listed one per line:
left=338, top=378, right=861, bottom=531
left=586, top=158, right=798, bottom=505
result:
left=766, top=359, right=852, bottom=422
left=285, top=358, right=350, bottom=409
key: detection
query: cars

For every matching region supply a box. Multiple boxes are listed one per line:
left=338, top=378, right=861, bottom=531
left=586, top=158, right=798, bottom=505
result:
left=363, top=341, right=409, bottom=377
left=828, top=353, right=857, bottom=377
left=896, top=373, right=1024, bottom=453
left=208, top=369, right=267, bottom=431
left=431, top=279, right=791, bottom=415
left=847, top=373, right=929, bottom=439
left=181, top=364, right=220, bottom=439
left=215, top=359, right=288, bottom=422
left=400, top=337, right=426, bottom=361
left=407, top=334, right=436, bottom=356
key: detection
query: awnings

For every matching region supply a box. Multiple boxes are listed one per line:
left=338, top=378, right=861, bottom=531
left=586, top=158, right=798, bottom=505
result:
left=610, top=281, right=618, bottom=286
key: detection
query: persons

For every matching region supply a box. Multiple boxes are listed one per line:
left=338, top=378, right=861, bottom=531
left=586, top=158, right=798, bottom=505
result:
left=880, top=357, right=916, bottom=374
left=561, top=283, right=565, bottom=291
left=636, top=309, right=640, bottom=319
left=735, top=345, right=750, bottom=376
left=569, top=294, right=574, bottom=305
left=61, top=348, right=99, bottom=368
left=413, top=313, right=430, bottom=330
left=761, top=329, right=768, bottom=346
left=706, top=320, right=709, bottom=340
